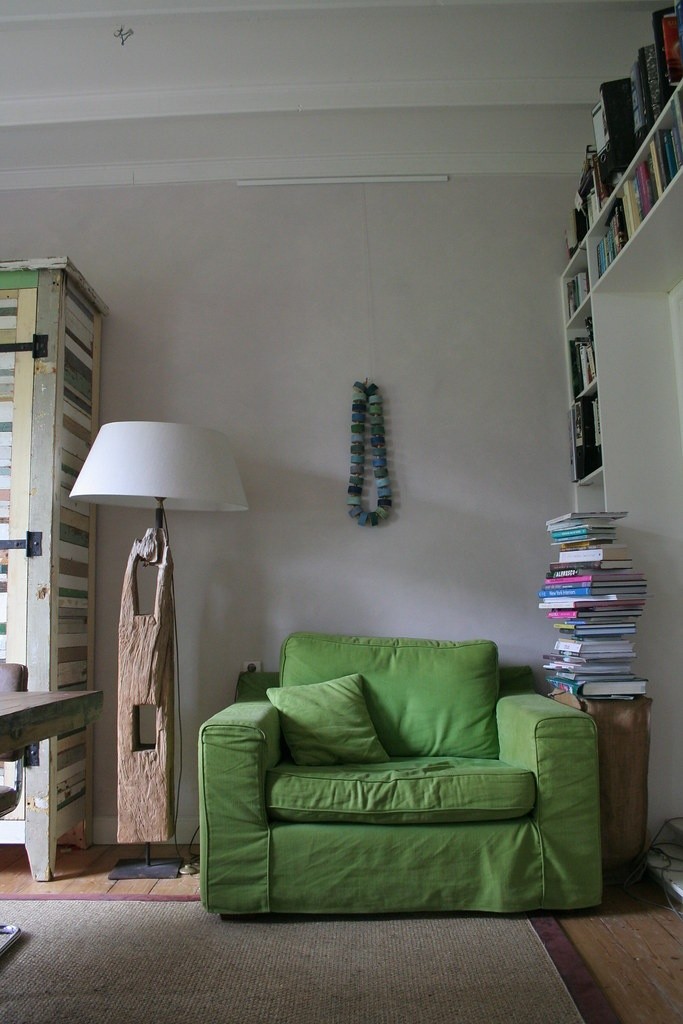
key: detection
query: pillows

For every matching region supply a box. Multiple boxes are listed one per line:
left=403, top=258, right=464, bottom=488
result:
left=267, top=673, right=392, bottom=766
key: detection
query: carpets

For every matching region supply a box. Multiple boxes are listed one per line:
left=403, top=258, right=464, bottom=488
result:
left=0, top=893, right=621, bottom=1024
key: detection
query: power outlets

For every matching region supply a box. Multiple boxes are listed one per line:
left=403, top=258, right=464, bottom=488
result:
left=243, top=661, right=261, bottom=673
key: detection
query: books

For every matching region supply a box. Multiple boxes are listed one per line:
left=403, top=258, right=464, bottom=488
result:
left=555, top=1, right=683, bottom=321
left=566, top=318, right=604, bottom=482
left=538, top=511, right=654, bottom=700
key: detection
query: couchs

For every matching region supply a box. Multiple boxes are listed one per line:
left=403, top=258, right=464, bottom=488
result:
left=198, top=633, right=604, bottom=918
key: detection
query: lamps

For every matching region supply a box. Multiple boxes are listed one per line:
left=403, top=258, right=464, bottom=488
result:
left=67, top=419, right=251, bottom=881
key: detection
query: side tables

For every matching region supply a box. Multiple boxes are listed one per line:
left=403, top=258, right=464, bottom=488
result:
left=548, top=687, right=654, bottom=889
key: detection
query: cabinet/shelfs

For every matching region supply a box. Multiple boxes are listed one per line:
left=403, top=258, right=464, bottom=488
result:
left=557, top=74, right=682, bottom=903
left=0, top=253, right=107, bottom=884
